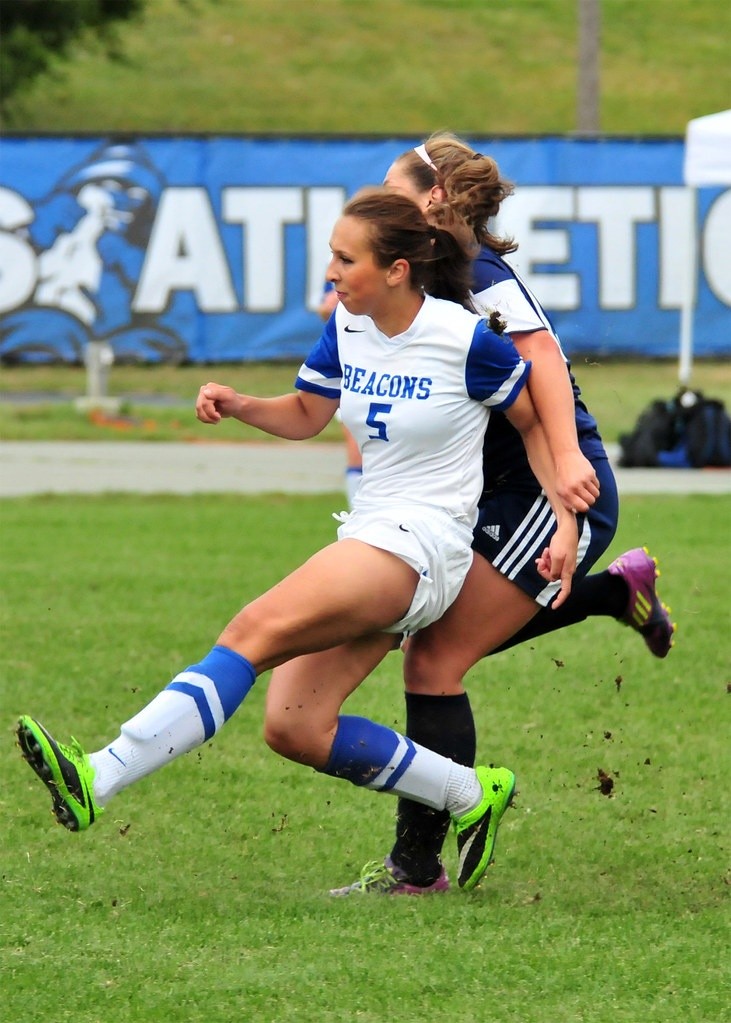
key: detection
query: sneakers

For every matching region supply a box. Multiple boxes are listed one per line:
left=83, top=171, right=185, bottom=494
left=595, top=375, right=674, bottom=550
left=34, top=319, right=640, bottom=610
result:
left=329, top=853, right=449, bottom=896
left=13, top=712, right=106, bottom=835
left=447, top=761, right=520, bottom=895
left=608, top=543, right=677, bottom=662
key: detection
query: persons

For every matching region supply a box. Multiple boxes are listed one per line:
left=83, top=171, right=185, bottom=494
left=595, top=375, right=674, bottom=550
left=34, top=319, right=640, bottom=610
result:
left=320, top=129, right=677, bottom=900
left=12, top=193, right=580, bottom=883
